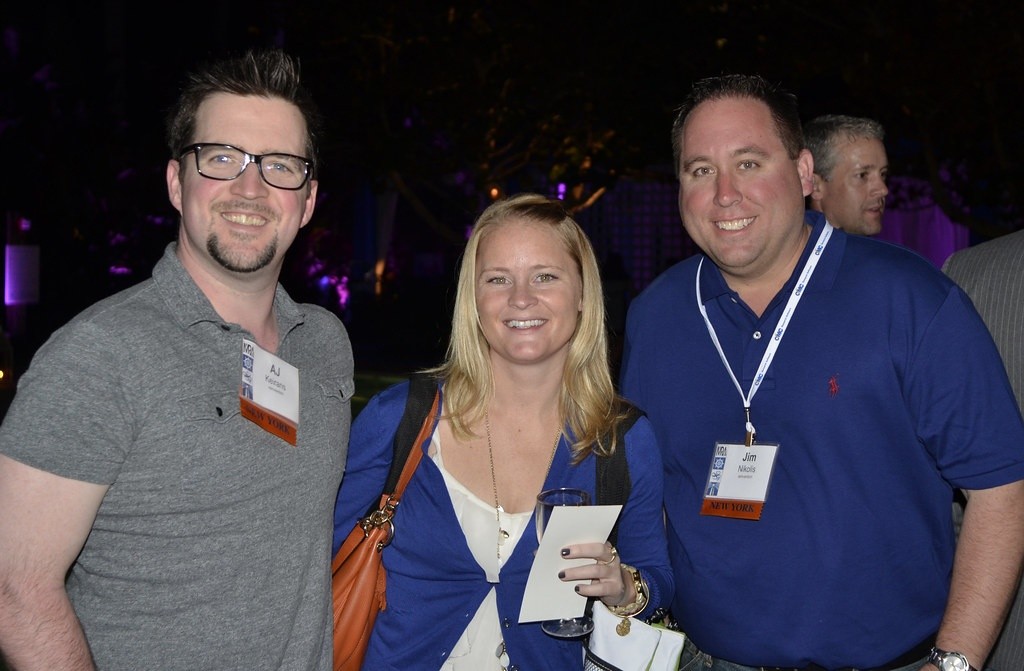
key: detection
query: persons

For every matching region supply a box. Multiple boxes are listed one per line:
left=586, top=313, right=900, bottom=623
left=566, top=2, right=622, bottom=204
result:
left=2, top=43, right=357, bottom=670
left=800, top=113, right=890, bottom=239
left=619, top=76, right=1024, bottom=670
left=330, top=196, right=675, bottom=669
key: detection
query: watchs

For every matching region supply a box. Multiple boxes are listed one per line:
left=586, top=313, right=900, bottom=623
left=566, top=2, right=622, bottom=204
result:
left=606, top=563, right=649, bottom=617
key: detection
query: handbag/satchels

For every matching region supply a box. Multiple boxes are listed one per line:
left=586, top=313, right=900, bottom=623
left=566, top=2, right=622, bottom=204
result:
left=333, top=390, right=439, bottom=671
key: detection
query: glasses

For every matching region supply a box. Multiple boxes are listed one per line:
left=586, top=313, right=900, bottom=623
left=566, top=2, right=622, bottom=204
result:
left=175, top=143, right=317, bottom=190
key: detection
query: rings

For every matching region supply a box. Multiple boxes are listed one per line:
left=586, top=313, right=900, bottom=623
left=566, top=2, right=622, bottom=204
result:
left=601, top=546, right=618, bottom=565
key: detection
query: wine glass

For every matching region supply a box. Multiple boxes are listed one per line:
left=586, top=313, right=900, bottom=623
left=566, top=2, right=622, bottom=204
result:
left=534, top=488, right=595, bottom=639
